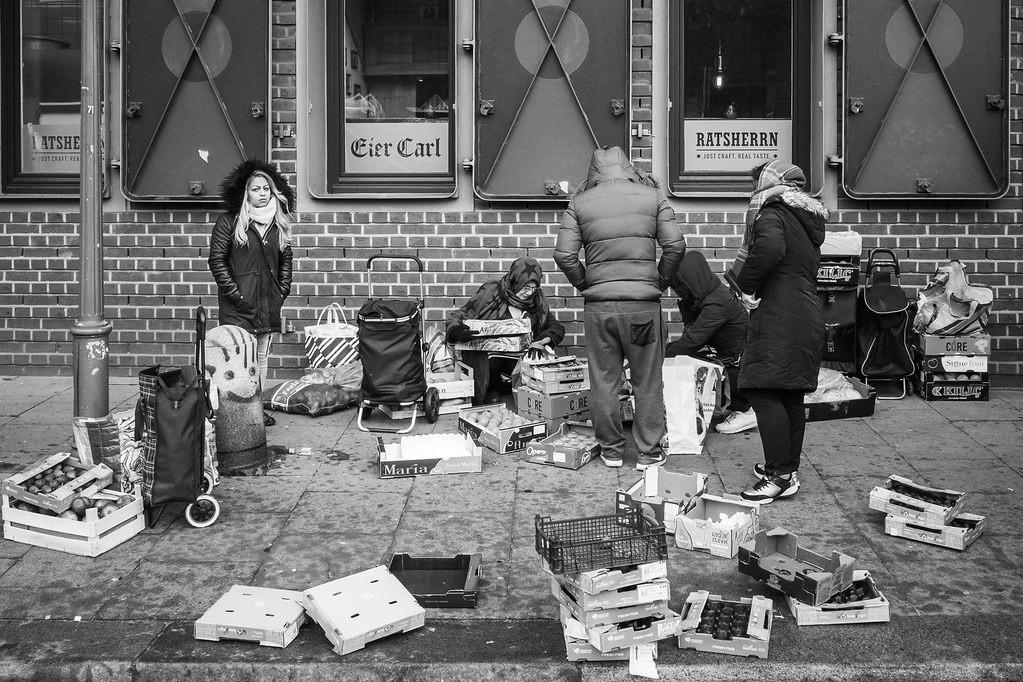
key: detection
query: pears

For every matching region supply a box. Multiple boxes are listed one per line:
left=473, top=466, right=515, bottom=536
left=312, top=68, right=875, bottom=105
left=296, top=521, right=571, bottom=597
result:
left=466, top=409, right=524, bottom=434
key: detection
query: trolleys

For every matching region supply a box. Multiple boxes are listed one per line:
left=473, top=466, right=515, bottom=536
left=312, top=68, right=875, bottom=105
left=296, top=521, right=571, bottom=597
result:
left=856, top=248, right=915, bottom=400
left=134, top=306, right=220, bottom=528
left=356, top=254, right=439, bottom=434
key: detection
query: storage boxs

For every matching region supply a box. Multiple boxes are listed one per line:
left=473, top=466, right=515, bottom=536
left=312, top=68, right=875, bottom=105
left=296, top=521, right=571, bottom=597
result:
left=2, top=449, right=144, bottom=558
left=194, top=551, right=481, bottom=655
left=454, top=402, right=544, bottom=452
left=526, top=420, right=603, bottom=470
left=516, top=410, right=593, bottom=436
left=802, top=375, right=876, bottom=420
left=375, top=434, right=485, bottom=478
left=519, top=357, right=590, bottom=392
left=916, top=330, right=992, bottom=401
left=534, top=465, right=986, bottom=662
left=514, top=386, right=591, bottom=416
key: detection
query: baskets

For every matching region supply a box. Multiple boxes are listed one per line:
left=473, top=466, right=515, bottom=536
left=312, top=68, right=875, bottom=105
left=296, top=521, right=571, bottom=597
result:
left=535, top=504, right=669, bottom=574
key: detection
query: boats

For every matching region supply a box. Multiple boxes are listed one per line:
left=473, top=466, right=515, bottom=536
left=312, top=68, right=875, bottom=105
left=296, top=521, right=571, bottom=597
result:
left=404, top=94, right=449, bottom=117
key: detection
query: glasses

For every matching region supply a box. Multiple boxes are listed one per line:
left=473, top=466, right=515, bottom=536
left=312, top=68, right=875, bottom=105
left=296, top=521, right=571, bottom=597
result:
left=522, top=287, right=539, bottom=292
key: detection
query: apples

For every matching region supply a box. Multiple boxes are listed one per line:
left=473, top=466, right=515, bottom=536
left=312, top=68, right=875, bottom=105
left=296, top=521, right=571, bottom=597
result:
left=17, top=465, right=134, bottom=521
left=265, top=381, right=361, bottom=417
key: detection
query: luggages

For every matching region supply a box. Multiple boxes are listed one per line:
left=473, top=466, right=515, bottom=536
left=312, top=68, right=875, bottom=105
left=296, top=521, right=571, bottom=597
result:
left=134, top=306, right=220, bottom=528
left=356, top=255, right=439, bottom=434
left=857, top=248, right=915, bottom=400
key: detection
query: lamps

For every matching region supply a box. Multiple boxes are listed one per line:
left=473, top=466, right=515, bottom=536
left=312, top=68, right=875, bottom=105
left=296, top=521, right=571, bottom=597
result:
left=710, top=40, right=730, bottom=89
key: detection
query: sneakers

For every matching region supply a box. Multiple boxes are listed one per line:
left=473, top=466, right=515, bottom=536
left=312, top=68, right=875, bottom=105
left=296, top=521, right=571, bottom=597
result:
left=715, top=406, right=758, bottom=433
left=752, top=462, right=801, bottom=487
left=739, top=472, right=798, bottom=505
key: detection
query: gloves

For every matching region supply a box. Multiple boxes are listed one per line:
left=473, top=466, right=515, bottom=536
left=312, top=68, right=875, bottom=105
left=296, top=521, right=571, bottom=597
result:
left=528, top=334, right=553, bottom=360
left=446, top=324, right=479, bottom=343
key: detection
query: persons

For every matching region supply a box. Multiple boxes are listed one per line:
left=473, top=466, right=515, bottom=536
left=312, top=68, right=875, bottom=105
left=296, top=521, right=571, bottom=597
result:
left=208, top=157, right=293, bottom=427
left=444, top=257, right=565, bottom=405
left=722, top=157, right=829, bottom=504
left=552, top=145, right=686, bottom=471
left=664, top=250, right=758, bottom=435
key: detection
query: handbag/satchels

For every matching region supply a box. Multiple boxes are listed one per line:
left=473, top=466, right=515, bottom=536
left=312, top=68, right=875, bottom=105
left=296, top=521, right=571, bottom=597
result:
left=306, top=303, right=359, bottom=370
left=911, top=260, right=993, bottom=336
left=624, top=355, right=731, bottom=456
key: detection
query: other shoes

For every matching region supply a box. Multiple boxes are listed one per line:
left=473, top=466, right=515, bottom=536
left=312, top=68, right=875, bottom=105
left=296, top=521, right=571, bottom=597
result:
left=600, top=453, right=623, bottom=467
left=636, top=453, right=666, bottom=471
left=264, top=412, right=276, bottom=426
